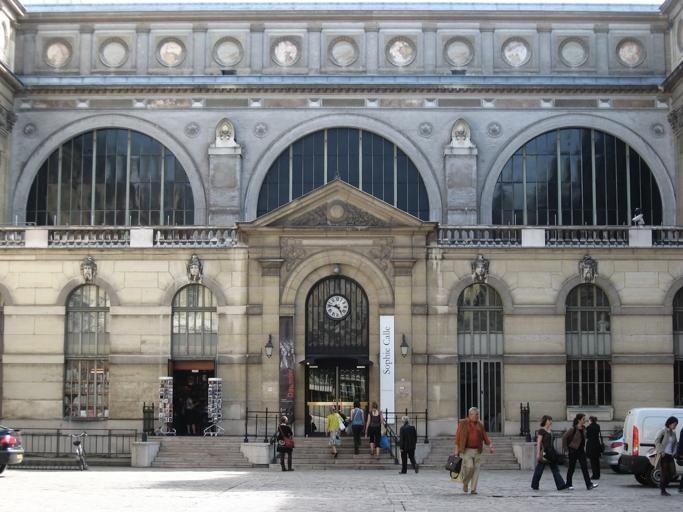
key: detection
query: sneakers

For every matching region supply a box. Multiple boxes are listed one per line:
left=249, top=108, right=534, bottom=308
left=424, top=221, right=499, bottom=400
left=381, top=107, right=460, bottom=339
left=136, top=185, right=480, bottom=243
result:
left=587, top=483, right=599, bottom=490
left=399, top=470, right=407, bottom=474
left=471, top=489, right=478, bottom=494
left=661, top=488, right=672, bottom=497
left=415, top=463, right=419, bottom=473
left=463, top=482, right=469, bottom=492
left=556, top=484, right=575, bottom=491
left=281, top=467, right=295, bottom=471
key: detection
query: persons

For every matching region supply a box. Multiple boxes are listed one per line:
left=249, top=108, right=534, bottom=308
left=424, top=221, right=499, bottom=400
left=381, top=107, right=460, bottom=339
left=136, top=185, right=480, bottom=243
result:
left=80, top=257, right=98, bottom=281
left=470, top=254, right=490, bottom=282
left=187, top=254, right=203, bottom=282
left=677, top=428, right=683, bottom=492
left=277, top=416, right=295, bottom=471
left=530, top=415, right=568, bottom=491
left=326, top=401, right=383, bottom=459
left=398, top=416, right=419, bottom=475
left=654, top=416, right=678, bottom=496
left=562, top=413, right=598, bottom=491
left=584, top=416, right=600, bottom=479
left=454, top=407, right=494, bottom=494
left=179, top=385, right=201, bottom=435
left=577, top=253, right=599, bottom=282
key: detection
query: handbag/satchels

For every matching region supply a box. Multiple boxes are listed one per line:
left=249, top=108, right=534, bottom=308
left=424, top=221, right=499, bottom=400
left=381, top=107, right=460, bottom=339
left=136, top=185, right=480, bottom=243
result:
left=284, top=437, right=295, bottom=450
left=600, top=442, right=605, bottom=453
left=444, top=454, right=464, bottom=482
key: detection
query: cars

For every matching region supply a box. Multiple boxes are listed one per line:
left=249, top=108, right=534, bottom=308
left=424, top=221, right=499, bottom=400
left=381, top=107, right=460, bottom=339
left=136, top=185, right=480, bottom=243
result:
left=0, top=425, right=23, bottom=477
left=603, top=426, right=629, bottom=474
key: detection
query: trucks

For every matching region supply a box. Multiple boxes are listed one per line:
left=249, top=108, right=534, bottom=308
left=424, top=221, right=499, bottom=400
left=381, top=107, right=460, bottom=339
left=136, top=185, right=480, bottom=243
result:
left=617, top=406, right=682, bottom=488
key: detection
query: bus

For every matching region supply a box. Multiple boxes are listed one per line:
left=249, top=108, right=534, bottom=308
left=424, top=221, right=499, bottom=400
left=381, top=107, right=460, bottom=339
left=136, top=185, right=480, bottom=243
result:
left=307, top=400, right=370, bottom=437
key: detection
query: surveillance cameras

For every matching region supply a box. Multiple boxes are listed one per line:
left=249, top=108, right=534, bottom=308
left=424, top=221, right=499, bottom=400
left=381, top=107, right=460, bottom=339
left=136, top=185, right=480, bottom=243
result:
left=632, top=214, right=643, bottom=222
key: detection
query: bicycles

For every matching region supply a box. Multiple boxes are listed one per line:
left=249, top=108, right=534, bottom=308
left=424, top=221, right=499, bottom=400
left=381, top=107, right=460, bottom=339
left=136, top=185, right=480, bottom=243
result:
left=66, top=431, right=89, bottom=471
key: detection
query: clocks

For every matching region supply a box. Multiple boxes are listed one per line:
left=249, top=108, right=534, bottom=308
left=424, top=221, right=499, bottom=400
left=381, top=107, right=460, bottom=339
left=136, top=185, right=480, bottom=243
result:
left=324, top=294, right=350, bottom=322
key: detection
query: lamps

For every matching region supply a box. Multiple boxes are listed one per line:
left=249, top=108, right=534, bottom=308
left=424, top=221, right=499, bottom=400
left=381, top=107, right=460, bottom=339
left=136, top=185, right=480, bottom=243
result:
left=399, top=333, right=409, bottom=359
left=263, top=333, right=273, bottom=361
